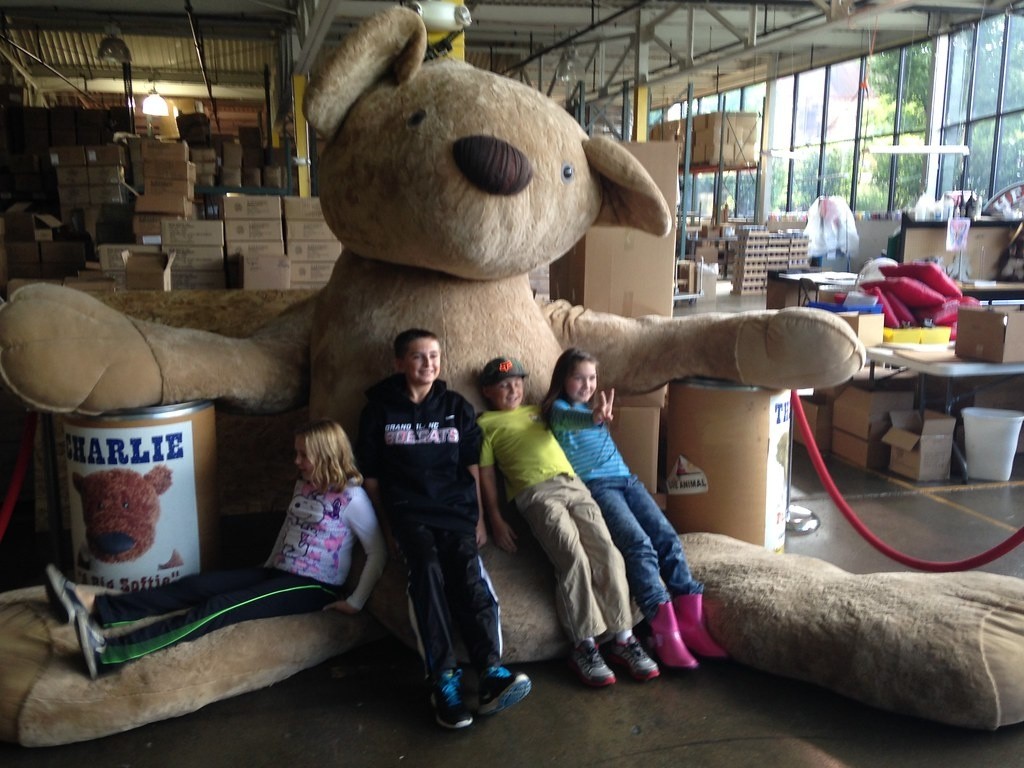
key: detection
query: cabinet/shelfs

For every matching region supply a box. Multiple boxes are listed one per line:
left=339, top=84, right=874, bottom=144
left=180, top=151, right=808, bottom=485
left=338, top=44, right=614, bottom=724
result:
left=574, top=78, right=765, bottom=310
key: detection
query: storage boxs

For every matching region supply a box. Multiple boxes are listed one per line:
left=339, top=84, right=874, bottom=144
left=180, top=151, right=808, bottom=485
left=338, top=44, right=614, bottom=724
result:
left=549, top=141, right=680, bottom=493
left=0, top=107, right=344, bottom=294
left=651, top=111, right=759, bottom=167
left=677, top=208, right=1024, bottom=481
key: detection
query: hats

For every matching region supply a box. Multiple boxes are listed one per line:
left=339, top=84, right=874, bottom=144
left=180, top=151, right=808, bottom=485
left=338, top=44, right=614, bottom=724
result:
left=482, top=356, right=530, bottom=392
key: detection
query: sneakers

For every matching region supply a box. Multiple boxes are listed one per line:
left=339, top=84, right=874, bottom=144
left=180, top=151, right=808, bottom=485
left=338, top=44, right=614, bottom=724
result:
left=46, top=564, right=88, bottom=624
left=75, top=612, right=109, bottom=679
left=478, top=666, right=532, bottom=714
left=426, top=668, right=472, bottom=728
left=610, top=634, right=660, bottom=679
left=573, top=639, right=617, bottom=685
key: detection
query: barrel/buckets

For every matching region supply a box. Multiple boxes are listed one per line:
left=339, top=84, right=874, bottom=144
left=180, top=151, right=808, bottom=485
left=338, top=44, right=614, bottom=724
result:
left=64, top=398, right=215, bottom=592
left=962, top=406, right=1024, bottom=481
left=668, top=377, right=788, bottom=554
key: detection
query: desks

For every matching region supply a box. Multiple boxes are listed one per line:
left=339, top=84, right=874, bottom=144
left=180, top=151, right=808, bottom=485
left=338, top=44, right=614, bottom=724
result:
left=865, top=346, right=1024, bottom=485
left=956, top=281, right=1024, bottom=311
left=776, top=270, right=859, bottom=287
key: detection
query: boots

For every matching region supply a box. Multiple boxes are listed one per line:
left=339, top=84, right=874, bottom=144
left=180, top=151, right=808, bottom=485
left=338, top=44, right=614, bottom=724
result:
left=672, top=593, right=728, bottom=660
left=649, top=601, right=700, bottom=668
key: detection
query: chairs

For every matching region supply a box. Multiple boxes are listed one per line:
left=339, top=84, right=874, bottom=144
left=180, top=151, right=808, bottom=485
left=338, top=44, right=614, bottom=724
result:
left=798, top=278, right=819, bottom=306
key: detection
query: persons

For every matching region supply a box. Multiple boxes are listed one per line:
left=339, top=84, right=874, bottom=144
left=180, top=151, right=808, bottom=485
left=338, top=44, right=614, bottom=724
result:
left=538, top=347, right=726, bottom=670
left=469, top=355, right=660, bottom=688
left=43, top=416, right=384, bottom=681
left=354, top=328, right=531, bottom=728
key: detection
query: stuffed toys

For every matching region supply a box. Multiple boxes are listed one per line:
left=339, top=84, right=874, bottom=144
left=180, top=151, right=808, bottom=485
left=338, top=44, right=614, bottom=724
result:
left=0, top=3, right=1024, bottom=747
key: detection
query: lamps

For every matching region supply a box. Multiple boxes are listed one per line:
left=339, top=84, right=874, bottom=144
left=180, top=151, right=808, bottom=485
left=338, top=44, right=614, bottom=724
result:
left=557, top=46, right=585, bottom=82
left=142, top=91, right=169, bottom=117
left=410, top=1, right=472, bottom=33
left=864, top=145, right=970, bottom=157
